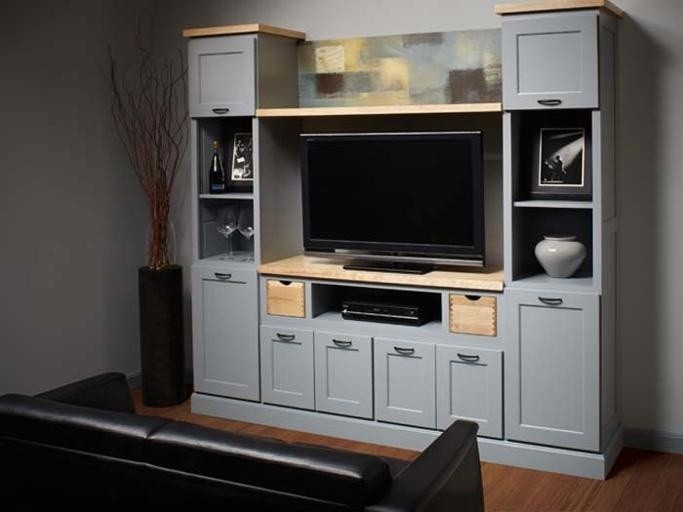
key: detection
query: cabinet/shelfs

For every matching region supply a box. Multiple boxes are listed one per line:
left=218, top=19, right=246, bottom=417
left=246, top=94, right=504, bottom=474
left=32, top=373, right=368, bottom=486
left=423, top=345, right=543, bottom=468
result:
left=503, top=0, right=623, bottom=452
left=258, top=279, right=311, bottom=422
left=436, top=291, right=504, bottom=443
left=313, top=311, right=436, bottom=433
left=182, top=22, right=306, bottom=404
left=254, top=102, right=502, bottom=294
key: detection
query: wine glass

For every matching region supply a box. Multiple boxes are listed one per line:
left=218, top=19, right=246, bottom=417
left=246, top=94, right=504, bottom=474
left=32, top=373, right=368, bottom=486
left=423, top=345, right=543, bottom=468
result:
left=216, top=208, right=237, bottom=262
left=238, top=208, right=254, bottom=262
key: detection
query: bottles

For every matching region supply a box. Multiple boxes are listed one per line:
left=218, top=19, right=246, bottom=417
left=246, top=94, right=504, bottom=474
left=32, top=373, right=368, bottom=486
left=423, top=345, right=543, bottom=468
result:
left=209, top=141, right=225, bottom=194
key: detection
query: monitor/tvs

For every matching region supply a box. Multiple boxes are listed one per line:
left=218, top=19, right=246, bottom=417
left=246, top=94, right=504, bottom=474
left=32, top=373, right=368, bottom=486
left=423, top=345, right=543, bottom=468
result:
left=296, top=130, right=487, bottom=276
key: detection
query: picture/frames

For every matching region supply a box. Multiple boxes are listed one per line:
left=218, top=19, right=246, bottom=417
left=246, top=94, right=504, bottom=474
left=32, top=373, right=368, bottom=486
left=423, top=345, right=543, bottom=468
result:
left=528, top=118, right=592, bottom=198
left=222, top=126, right=253, bottom=192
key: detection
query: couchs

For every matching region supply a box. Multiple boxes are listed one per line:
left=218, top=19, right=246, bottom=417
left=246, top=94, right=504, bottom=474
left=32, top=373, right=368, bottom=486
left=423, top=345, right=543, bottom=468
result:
left=0, top=371, right=486, bottom=511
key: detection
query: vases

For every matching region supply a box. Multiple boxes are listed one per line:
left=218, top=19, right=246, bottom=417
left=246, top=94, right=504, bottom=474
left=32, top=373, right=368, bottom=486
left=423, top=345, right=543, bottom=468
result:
left=535, top=235, right=587, bottom=279
left=139, top=264, right=185, bottom=407
